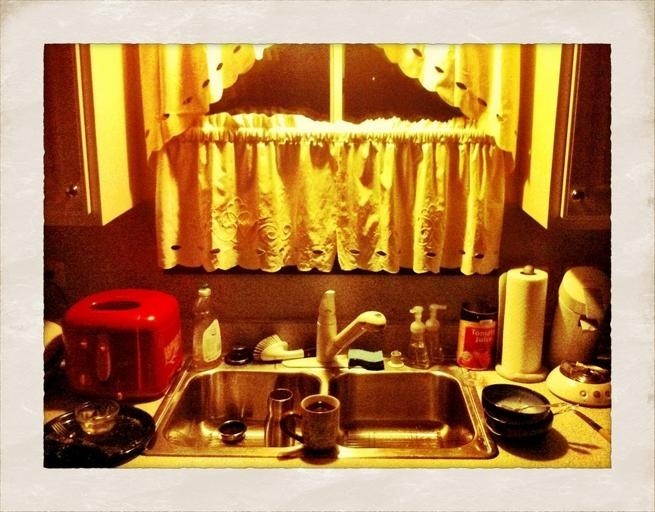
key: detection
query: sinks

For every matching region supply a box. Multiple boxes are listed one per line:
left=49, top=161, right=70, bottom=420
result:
left=141, top=367, right=323, bottom=460
left=323, top=368, right=498, bottom=460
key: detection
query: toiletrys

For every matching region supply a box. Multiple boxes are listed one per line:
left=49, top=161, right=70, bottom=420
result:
left=406, top=306, right=431, bottom=367
left=424, top=304, right=448, bottom=364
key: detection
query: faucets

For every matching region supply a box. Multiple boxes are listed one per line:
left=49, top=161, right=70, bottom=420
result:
left=314, top=288, right=389, bottom=369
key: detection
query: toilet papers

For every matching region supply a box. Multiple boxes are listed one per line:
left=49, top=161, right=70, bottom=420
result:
left=497, top=268, right=547, bottom=374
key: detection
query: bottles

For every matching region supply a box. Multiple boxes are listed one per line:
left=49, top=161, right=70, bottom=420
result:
left=550, top=267, right=611, bottom=366
left=264, top=389, right=296, bottom=448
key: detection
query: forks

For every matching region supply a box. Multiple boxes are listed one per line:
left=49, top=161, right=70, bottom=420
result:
left=51, top=422, right=123, bottom=453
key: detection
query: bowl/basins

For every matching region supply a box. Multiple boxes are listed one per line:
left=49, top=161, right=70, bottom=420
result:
left=482, top=384, right=554, bottom=444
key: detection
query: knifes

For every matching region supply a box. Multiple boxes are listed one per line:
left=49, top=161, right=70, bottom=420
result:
left=573, top=410, right=611, bottom=443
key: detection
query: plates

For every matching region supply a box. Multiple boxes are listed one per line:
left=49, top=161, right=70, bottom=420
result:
left=44, top=404, right=155, bottom=468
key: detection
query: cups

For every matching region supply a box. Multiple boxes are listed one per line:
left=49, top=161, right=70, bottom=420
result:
left=75, top=400, right=120, bottom=435
left=279, top=394, right=341, bottom=451
left=217, top=420, right=246, bottom=442
